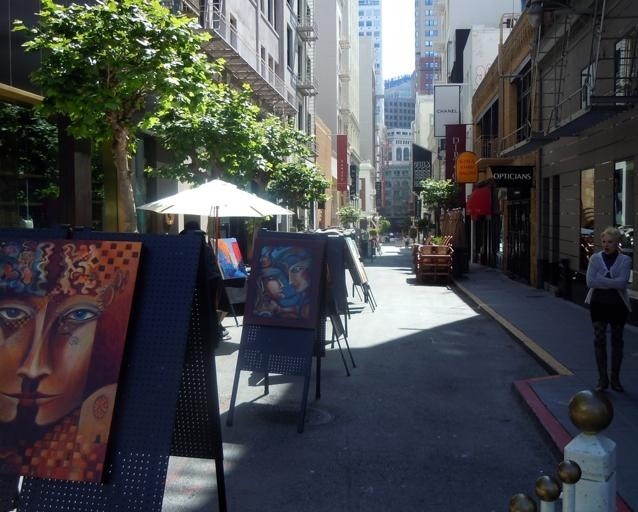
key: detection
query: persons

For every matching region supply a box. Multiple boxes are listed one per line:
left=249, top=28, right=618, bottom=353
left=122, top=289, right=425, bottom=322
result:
left=584, top=227, right=633, bottom=394
left=0, top=238, right=142, bottom=481
left=253, top=244, right=313, bottom=322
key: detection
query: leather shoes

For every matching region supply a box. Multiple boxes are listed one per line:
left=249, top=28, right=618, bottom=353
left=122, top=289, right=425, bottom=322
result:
left=594, top=383, right=608, bottom=392
left=611, top=380, right=624, bottom=392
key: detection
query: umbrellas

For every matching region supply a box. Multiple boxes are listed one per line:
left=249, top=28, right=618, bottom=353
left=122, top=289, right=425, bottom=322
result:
left=135, top=179, right=295, bottom=310
